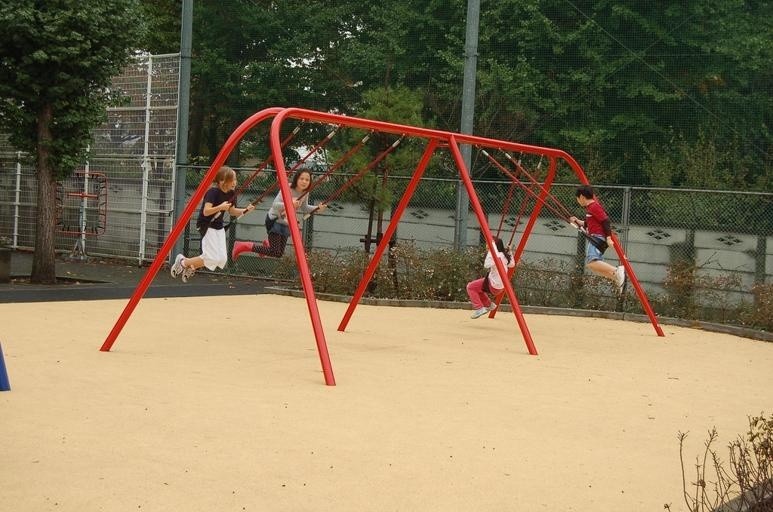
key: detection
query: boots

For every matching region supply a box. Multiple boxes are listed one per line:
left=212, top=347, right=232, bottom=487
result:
left=230, top=240, right=254, bottom=262
left=258, top=239, right=268, bottom=258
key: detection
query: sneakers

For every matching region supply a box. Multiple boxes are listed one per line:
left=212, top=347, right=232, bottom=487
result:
left=181, top=268, right=194, bottom=283
left=614, top=265, right=625, bottom=287
left=614, top=283, right=624, bottom=294
left=170, top=253, right=185, bottom=279
left=470, top=306, right=487, bottom=320
left=486, top=302, right=497, bottom=313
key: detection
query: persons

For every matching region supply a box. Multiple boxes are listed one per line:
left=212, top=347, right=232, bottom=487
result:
left=229, top=167, right=328, bottom=263
left=568, top=184, right=625, bottom=294
left=167, top=166, right=256, bottom=284
left=466, top=236, right=515, bottom=319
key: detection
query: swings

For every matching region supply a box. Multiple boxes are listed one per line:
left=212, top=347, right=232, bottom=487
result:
left=481, top=147, right=609, bottom=254
left=481, top=152, right=544, bottom=294
left=267, top=129, right=406, bottom=235
left=197, top=118, right=343, bottom=235
